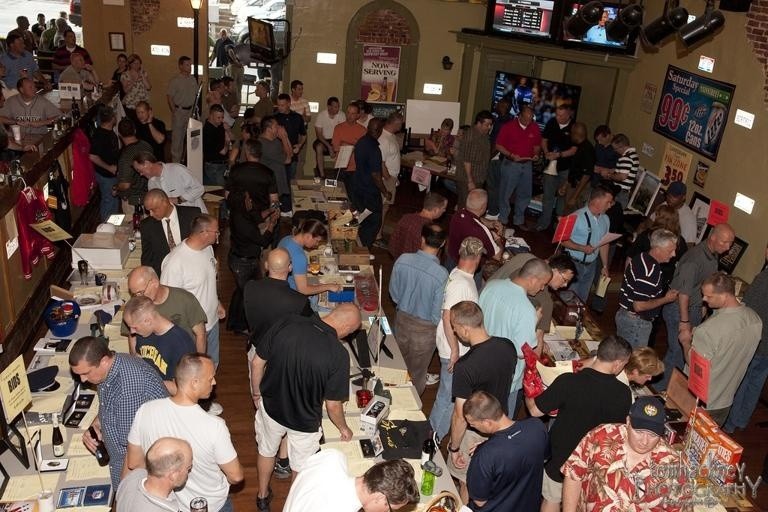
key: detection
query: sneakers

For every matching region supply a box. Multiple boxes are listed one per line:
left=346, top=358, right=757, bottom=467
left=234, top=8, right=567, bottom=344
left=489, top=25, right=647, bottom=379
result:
left=426, top=373, right=440, bottom=385
left=485, top=212, right=500, bottom=221
left=372, top=239, right=387, bottom=249
left=532, top=224, right=547, bottom=232
left=273, top=462, right=292, bottom=477
left=257, top=488, right=272, bottom=510
left=200, top=401, right=223, bottom=416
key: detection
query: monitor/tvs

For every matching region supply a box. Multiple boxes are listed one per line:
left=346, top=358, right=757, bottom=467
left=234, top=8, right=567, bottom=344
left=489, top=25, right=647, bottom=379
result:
left=558, top=0, right=641, bottom=56
left=490, top=69, right=583, bottom=132
left=247, top=17, right=276, bottom=63
left=482, top=0, right=553, bottom=43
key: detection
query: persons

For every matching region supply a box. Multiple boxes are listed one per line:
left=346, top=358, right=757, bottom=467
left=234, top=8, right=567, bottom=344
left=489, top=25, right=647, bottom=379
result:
left=524, top=333, right=633, bottom=511
left=249, top=301, right=361, bottom=512
left=557, top=394, right=694, bottom=512
left=114, top=437, right=194, bottom=512
left=461, top=389, right=552, bottom=511
left=0, top=9, right=767, bottom=435
left=68, top=335, right=174, bottom=492
left=281, top=449, right=419, bottom=512
left=446, top=299, right=518, bottom=505
left=126, top=351, right=245, bottom=512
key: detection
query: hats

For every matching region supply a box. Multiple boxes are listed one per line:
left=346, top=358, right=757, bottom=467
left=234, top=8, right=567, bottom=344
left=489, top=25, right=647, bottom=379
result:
left=28, top=366, right=60, bottom=393
left=460, top=237, right=488, bottom=255
left=666, top=181, right=686, bottom=196
left=629, top=397, right=665, bottom=434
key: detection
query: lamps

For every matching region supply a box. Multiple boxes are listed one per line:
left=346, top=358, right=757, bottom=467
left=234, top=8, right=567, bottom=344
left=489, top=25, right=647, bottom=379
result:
left=566, top=1, right=725, bottom=49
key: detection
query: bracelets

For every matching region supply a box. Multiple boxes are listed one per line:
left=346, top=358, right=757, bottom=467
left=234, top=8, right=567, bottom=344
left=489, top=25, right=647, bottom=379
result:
left=446, top=442, right=460, bottom=453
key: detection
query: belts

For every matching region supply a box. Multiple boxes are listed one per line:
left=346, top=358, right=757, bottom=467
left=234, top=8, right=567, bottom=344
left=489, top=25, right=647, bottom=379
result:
left=229, top=248, right=259, bottom=259
left=175, top=105, right=193, bottom=110
left=570, top=256, right=591, bottom=266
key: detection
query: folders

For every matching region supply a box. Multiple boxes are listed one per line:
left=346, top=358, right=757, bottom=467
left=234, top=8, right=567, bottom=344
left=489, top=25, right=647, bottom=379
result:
left=43, top=338, right=73, bottom=352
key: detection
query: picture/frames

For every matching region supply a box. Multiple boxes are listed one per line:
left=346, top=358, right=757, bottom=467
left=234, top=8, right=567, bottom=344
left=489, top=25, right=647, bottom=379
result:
left=653, top=63, right=737, bottom=162
left=108, top=32, right=126, bottom=51
left=361, top=44, right=402, bottom=104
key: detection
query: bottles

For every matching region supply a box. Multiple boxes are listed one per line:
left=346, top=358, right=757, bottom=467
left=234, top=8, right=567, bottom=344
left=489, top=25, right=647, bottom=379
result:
left=89, top=426, right=110, bottom=467
left=71, top=96, right=81, bottom=120
left=420, top=428, right=435, bottom=470
left=420, top=460, right=443, bottom=496
left=346, top=276, right=353, bottom=284
left=99, top=81, right=104, bottom=94
left=133, top=197, right=144, bottom=230
left=51, top=413, right=65, bottom=458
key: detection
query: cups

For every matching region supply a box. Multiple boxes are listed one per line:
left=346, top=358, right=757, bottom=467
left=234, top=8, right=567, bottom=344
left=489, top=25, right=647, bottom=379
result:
left=95, top=273, right=107, bottom=286
left=310, top=258, right=320, bottom=274
left=78, top=259, right=89, bottom=279
left=12, top=125, right=21, bottom=140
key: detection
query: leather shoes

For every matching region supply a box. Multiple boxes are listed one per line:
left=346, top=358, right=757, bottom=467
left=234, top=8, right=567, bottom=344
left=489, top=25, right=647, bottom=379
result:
left=517, top=223, right=528, bottom=231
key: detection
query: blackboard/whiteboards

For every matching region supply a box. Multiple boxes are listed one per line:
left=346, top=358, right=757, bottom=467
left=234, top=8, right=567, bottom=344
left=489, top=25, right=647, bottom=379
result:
left=405, top=99, right=460, bottom=136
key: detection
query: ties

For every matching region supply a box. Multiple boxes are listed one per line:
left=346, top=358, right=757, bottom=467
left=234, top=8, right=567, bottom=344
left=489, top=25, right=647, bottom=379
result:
left=166, top=219, right=176, bottom=251
